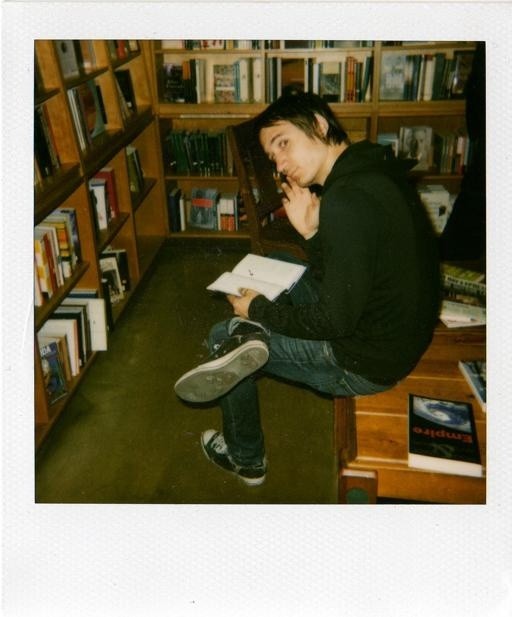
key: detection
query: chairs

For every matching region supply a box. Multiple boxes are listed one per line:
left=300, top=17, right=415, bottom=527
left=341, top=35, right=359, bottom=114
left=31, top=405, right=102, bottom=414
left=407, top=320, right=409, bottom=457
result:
left=226, top=117, right=309, bottom=261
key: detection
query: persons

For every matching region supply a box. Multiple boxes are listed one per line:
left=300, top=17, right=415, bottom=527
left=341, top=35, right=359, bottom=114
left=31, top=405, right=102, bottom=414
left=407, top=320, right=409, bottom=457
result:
left=170, top=92, right=443, bottom=486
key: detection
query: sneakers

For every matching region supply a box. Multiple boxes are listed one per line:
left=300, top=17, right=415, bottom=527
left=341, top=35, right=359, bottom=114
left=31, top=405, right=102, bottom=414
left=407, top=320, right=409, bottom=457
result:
left=173, top=329, right=270, bottom=403
left=199, top=428, right=268, bottom=487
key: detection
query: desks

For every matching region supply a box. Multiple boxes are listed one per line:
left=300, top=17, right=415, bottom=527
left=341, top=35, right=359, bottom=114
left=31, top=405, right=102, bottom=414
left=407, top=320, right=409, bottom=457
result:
left=333, top=317, right=486, bottom=503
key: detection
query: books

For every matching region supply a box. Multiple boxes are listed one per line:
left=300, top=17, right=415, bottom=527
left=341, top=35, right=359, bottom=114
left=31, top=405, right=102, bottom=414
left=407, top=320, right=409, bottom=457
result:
left=439, top=262, right=486, bottom=328
left=457, top=357, right=486, bottom=414
left=407, top=392, right=483, bottom=478
left=207, top=252, right=307, bottom=300
left=35, top=40, right=147, bottom=412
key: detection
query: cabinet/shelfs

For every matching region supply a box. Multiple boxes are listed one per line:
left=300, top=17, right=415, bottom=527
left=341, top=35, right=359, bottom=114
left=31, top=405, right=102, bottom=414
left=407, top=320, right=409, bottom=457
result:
left=34, top=39, right=167, bottom=455
